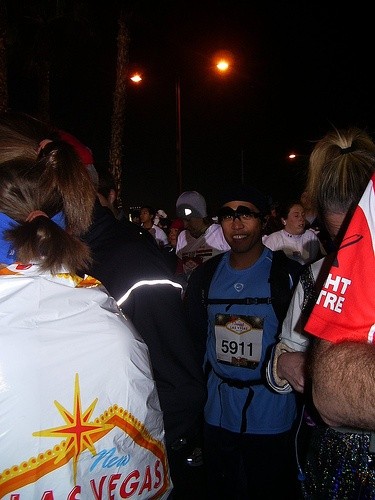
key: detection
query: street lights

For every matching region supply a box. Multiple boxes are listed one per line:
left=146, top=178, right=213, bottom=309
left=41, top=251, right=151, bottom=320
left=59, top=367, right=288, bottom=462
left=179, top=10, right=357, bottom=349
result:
left=130, top=52, right=232, bottom=182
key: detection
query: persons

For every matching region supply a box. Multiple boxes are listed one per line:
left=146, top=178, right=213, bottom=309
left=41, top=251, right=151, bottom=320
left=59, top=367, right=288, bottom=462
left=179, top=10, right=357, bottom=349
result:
left=0, top=111, right=375, bottom=500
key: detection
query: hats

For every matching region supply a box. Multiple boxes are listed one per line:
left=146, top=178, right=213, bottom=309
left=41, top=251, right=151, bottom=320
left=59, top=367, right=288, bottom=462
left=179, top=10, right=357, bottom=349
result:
left=59, top=129, right=94, bottom=165
left=219, top=185, right=265, bottom=212
left=176, top=190, right=208, bottom=219
left=170, top=218, right=184, bottom=231
